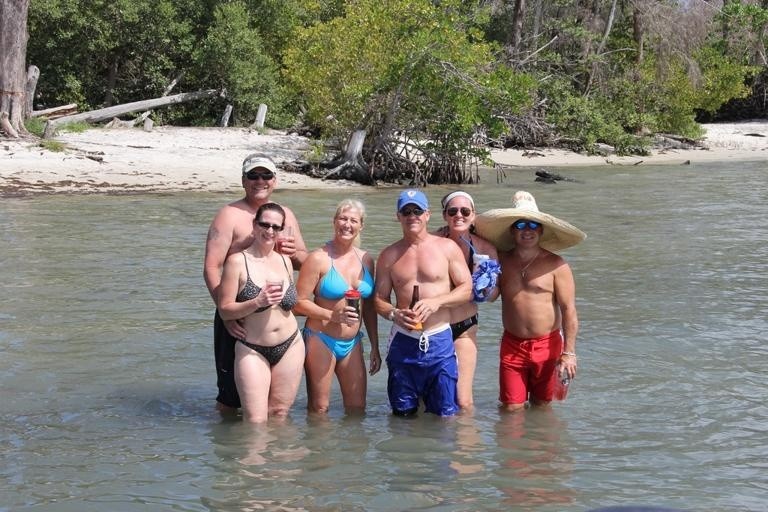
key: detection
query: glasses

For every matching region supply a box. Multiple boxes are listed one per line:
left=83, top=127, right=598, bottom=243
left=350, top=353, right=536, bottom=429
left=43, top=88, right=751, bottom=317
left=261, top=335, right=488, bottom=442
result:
left=399, top=207, right=426, bottom=216
left=243, top=172, right=273, bottom=180
left=445, top=207, right=473, bottom=217
left=255, top=219, right=283, bottom=232
left=512, top=221, right=541, bottom=230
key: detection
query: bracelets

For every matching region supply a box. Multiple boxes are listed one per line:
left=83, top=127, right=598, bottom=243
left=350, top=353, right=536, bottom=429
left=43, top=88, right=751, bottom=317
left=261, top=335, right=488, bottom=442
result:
left=561, top=351, right=576, bottom=356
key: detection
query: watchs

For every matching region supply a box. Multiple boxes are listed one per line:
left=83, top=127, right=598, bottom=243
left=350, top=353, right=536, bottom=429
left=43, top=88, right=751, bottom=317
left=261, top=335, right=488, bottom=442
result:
left=388, top=308, right=400, bottom=320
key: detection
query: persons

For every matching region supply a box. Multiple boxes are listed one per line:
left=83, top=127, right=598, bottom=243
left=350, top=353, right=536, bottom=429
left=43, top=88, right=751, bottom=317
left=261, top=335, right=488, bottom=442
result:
left=472, top=219, right=579, bottom=413
left=427, top=190, right=500, bottom=414
left=218, top=204, right=306, bottom=422
left=203, top=152, right=310, bottom=414
left=292, top=197, right=382, bottom=415
left=370, top=189, right=474, bottom=417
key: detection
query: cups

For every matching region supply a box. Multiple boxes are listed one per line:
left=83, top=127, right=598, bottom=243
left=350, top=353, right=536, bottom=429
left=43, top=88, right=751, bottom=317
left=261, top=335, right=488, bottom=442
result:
left=275, top=227, right=294, bottom=254
left=556, top=364, right=569, bottom=385
left=472, top=253, right=490, bottom=274
left=345, top=290, right=360, bottom=319
left=266, top=279, right=284, bottom=301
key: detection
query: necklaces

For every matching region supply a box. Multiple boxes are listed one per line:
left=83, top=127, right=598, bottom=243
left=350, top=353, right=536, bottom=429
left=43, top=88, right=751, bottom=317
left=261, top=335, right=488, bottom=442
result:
left=513, top=247, right=541, bottom=278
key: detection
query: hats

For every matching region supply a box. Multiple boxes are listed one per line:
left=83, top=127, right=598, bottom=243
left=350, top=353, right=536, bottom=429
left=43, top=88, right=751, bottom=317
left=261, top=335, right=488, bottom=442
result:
left=475, top=192, right=587, bottom=252
left=399, top=190, right=428, bottom=212
left=243, top=154, right=277, bottom=175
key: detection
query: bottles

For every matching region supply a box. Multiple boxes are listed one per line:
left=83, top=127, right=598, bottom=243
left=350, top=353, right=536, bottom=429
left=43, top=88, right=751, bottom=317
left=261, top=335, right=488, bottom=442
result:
left=408, top=284, right=423, bottom=331
